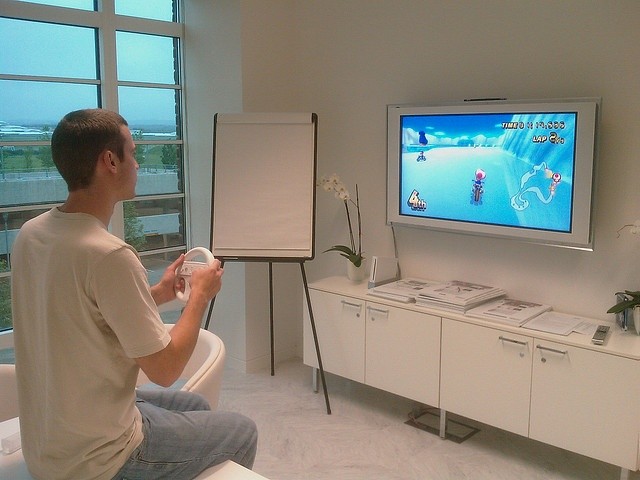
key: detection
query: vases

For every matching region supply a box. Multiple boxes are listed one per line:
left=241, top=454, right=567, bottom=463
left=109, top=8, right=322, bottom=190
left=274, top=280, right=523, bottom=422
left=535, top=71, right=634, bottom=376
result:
left=345, top=259, right=365, bottom=282
left=631, top=306, right=639, bottom=337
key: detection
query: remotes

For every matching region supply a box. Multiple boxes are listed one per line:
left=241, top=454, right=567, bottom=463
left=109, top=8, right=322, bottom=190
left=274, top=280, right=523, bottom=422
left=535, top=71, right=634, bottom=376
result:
left=591, top=324, right=612, bottom=346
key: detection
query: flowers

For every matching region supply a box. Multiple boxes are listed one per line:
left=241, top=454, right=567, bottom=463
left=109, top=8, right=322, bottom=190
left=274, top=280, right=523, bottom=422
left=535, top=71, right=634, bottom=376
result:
left=605, top=221, right=640, bottom=315
left=319, top=169, right=363, bottom=268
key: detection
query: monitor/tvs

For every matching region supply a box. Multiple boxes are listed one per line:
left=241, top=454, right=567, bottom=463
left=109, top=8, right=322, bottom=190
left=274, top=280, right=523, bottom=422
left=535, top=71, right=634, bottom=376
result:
left=385, top=96, right=602, bottom=253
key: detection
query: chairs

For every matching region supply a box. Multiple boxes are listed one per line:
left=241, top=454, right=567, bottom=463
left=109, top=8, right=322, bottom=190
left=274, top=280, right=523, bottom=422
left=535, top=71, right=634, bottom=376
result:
left=135, top=321, right=227, bottom=410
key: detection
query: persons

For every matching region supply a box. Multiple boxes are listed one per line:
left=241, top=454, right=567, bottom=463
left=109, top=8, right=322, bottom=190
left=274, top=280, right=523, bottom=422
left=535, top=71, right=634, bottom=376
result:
left=8, top=107, right=259, bottom=479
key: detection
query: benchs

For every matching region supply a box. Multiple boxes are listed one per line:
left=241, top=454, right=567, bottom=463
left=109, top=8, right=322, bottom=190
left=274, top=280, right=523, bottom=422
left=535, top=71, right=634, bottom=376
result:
left=1, top=407, right=275, bottom=480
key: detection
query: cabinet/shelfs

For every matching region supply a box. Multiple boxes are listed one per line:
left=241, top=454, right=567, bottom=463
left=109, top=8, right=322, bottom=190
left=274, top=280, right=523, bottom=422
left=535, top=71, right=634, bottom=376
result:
left=300, top=275, right=442, bottom=480
left=438, top=286, right=638, bottom=476
